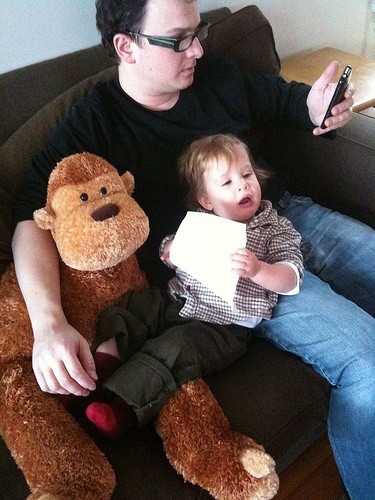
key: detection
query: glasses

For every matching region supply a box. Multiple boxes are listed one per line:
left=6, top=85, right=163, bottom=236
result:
left=124, top=21, right=211, bottom=53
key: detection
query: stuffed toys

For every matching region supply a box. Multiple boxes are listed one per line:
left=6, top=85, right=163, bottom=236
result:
left=0, top=151, right=282, bottom=500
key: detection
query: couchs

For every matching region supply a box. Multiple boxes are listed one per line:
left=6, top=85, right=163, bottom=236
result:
left=0, top=5, right=375, bottom=500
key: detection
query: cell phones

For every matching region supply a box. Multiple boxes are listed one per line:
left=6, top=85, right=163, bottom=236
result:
left=318, top=65, right=352, bottom=139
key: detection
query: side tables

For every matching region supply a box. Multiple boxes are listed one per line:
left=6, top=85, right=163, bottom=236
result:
left=279, top=45, right=375, bottom=116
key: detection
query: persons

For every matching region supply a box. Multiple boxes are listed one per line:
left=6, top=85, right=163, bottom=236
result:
left=77, top=134, right=306, bottom=435
left=6, top=0, right=375, bottom=500
